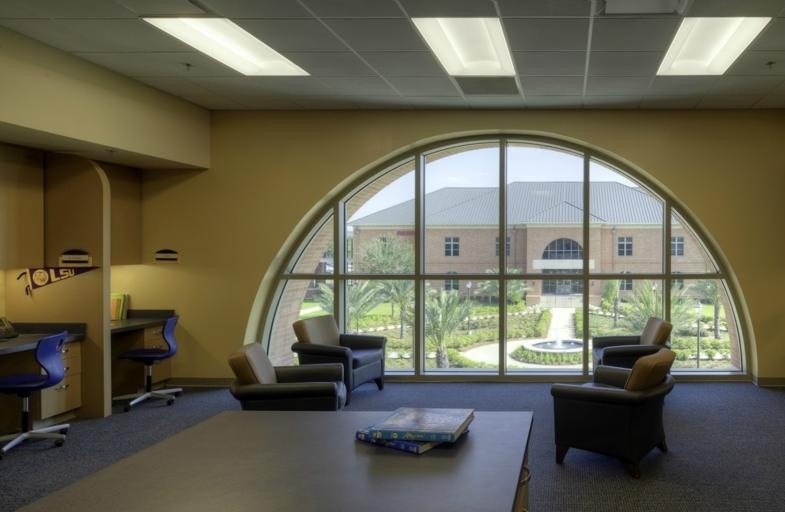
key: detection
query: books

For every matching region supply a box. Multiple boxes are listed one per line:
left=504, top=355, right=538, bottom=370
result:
left=110, top=292, right=125, bottom=320
left=354, top=422, right=469, bottom=456
left=122, top=294, right=129, bottom=320
left=371, top=405, right=474, bottom=442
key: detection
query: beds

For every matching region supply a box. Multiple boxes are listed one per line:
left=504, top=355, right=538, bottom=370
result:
left=550, top=315, right=677, bottom=480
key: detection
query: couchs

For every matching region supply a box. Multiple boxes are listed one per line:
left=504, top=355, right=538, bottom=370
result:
left=227, top=314, right=387, bottom=410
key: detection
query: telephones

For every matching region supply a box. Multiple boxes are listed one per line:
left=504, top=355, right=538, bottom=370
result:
left=0, top=317, right=18, bottom=339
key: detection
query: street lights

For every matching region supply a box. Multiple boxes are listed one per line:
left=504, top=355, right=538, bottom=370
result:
left=465, top=280, right=473, bottom=305
left=652, top=282, right=660, bottom=316
left=693, top=299, right=706, bottom=369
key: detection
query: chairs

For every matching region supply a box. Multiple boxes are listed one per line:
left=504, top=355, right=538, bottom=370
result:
left=112, top=315, right=184, bottom=413
left=1, top=330, right=70, bottom=456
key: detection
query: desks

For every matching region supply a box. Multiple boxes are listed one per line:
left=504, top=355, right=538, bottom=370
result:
left=0, top=322, right=87, bottom=431
left=110, top=308, right=176, bottom=394
left=6, top=408, right=536, bottom=512
